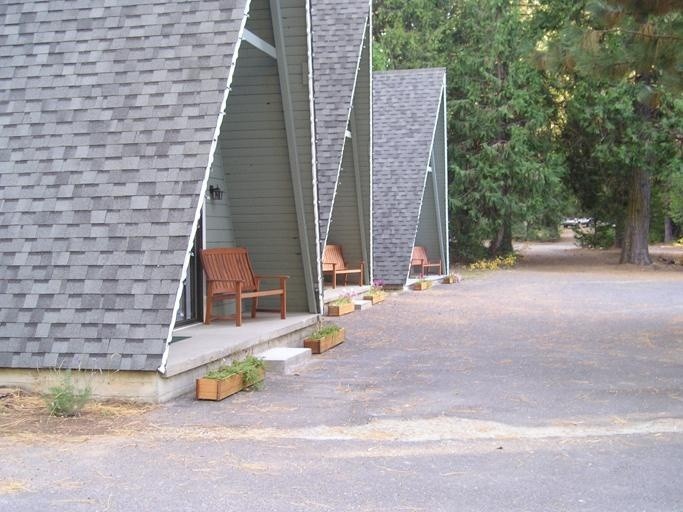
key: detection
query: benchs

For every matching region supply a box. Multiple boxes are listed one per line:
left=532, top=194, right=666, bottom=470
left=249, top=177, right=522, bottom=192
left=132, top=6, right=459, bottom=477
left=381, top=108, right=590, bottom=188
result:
left=320, top=243, right=365, bottom=290
left=198, top=247, right=290, bottom=327
left=408, top=246, right=442, bottom=279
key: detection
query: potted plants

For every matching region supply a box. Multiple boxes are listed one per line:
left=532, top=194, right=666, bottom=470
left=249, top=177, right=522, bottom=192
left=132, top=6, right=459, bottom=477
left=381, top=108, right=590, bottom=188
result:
left=302, top=318, right=345, bottom=355
left=194, top=352, right=268, bottom=403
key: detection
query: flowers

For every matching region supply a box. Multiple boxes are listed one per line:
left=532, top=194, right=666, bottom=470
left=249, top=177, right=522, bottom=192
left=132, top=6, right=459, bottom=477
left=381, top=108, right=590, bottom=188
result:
left=416, top=272, right=430, bottom=283
left=331, top=278, right=384, bottom=304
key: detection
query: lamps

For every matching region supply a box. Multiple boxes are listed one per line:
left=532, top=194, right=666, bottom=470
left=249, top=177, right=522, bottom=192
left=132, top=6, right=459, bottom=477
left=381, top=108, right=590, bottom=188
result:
left=208, top=183, right=222, bottom=201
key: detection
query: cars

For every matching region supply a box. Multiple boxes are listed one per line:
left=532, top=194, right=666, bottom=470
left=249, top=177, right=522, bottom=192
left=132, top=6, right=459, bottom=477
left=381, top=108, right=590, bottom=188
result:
left=564, top=217, right=591, bottom=228
